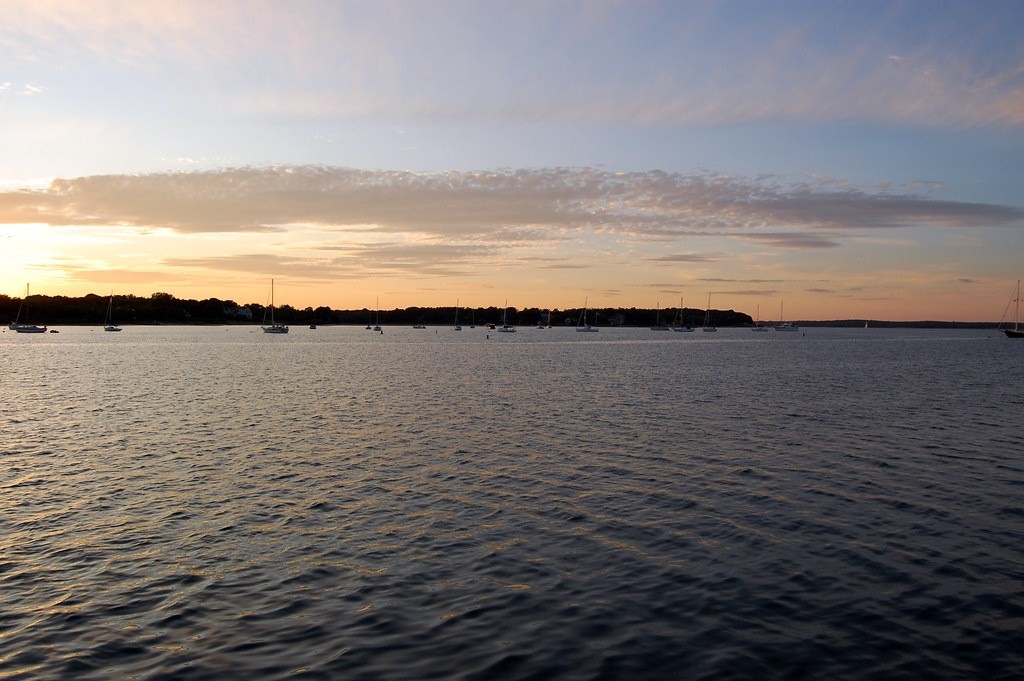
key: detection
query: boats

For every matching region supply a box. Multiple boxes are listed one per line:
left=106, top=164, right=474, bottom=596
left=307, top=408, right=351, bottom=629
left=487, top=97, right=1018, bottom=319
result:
left=16, top=326, right=46, bottom=334
left=489, top=323, right=495, bottom=330
left=536, top=325, right=544, bottom=329
left=412, top=324, right=425, bottom=329
left=310, top=325, right=317, bottom=328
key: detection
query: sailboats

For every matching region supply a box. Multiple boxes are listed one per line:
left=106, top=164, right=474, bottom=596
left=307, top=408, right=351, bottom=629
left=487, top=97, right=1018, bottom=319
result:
left=703, top=292, right=718, bottom=332
left=751, top=304, right=769, bottom=332
left=547, top=309, right=552, bottom=329
left=469, top=305, right=476, bottom=328
left=454, top=298, right=462, bottom=330
left=672, top=298, right=695, bottom=332
left=773, top=300, right=798, bottom=332
left=259, top=277, right=289, bottom=334
left=365, top=312, right=372, bottom=329
left=994, top=278, right=1024, bottom=338
left=497, top=298, right=517, bottom=333
left=374, top=296, right=381, bottom=331
left=105, top=293, right=122, bottom=331
left=10, top=283, right=30, bottom=331
left=575, top=297, right=599, bottom=333
left=648, top=301, right=670, bottom=331
